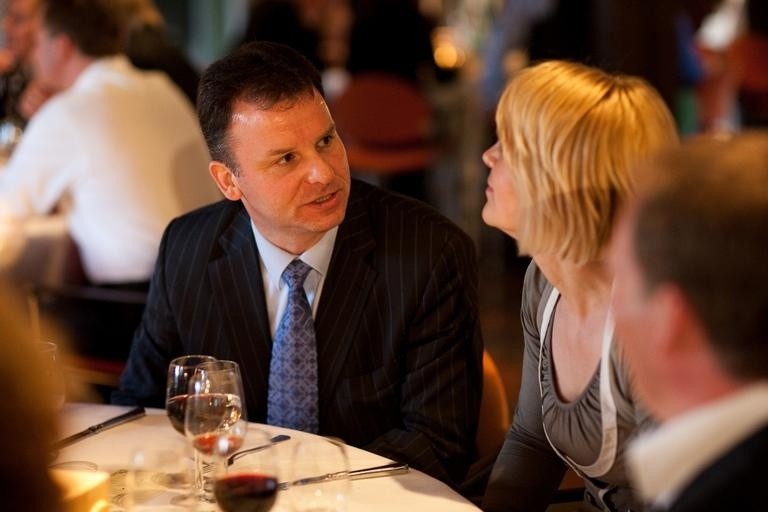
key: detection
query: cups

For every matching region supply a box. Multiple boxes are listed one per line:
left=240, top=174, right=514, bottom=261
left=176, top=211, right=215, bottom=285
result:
left=208, top=426, right=280, bottom=511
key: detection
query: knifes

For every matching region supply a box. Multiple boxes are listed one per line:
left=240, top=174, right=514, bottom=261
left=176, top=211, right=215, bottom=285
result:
left=35, top=405, right=150, bottom=453
left=274, top=458, right=411, bottom=489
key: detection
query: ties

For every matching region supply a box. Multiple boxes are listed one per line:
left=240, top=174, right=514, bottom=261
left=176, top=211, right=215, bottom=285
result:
left=267, top=260, right=320, bottom=434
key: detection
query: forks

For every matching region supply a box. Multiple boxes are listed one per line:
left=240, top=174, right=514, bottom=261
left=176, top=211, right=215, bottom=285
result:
left=187, top=434, right=294, bottom=475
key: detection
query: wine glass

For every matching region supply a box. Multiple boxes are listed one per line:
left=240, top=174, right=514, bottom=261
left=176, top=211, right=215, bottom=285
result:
left=165, top=354, right=229, bottom=492
left=35, top=340, right=67, bottom=466
left=170, top=357, right=250, bottom=508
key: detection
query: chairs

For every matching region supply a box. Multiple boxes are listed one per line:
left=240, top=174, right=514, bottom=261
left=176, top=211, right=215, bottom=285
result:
left=474, top=350, right=507, bottom=464
left=22, top=281, right=149, bottom=391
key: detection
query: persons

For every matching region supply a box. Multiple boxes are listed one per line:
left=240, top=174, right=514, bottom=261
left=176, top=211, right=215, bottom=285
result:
left=477, top=59, right=685, bottom=510
left=112, top=37, right=485, bottom=489
left=599, top=124, right=766, bottom=510
left=1, top=275, right=83, bottom=512
left=1, top=1, right=768, bottom=361
